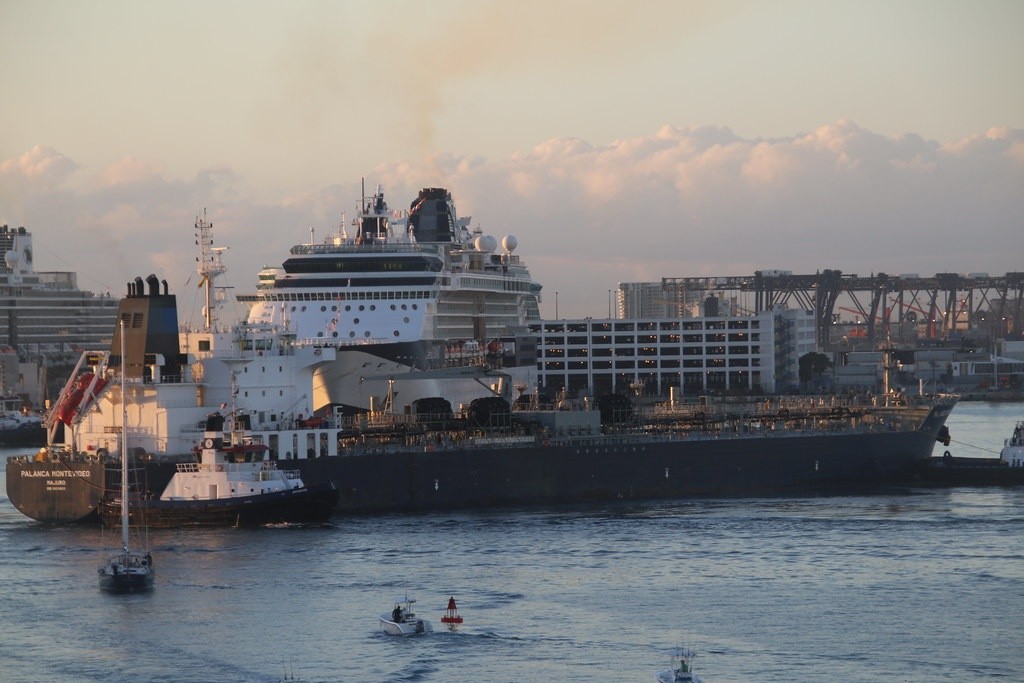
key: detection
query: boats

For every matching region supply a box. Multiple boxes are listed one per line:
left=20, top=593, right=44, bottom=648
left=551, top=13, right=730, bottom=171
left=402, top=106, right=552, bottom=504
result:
left=160, top=371, right=311, bottom=525
left=0, top=225, right=188, bottom=448
left=378, top=596, right=435, bottom=637
left=917, top=421, right=1024, bottom=481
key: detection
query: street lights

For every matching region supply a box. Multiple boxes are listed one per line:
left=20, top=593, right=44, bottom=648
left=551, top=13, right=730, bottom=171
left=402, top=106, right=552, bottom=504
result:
left=555, top=292, right=558, bottom=319
left=608, top=289, right=611, bottom=318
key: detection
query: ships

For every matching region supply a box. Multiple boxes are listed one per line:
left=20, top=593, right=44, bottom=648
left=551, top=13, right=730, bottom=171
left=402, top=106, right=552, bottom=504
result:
left=5, top=177, right=966, bottom=526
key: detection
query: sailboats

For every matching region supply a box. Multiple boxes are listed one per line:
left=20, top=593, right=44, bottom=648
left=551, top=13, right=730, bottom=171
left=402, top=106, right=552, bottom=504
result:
left=95, top=319, right=155, bottom=594
